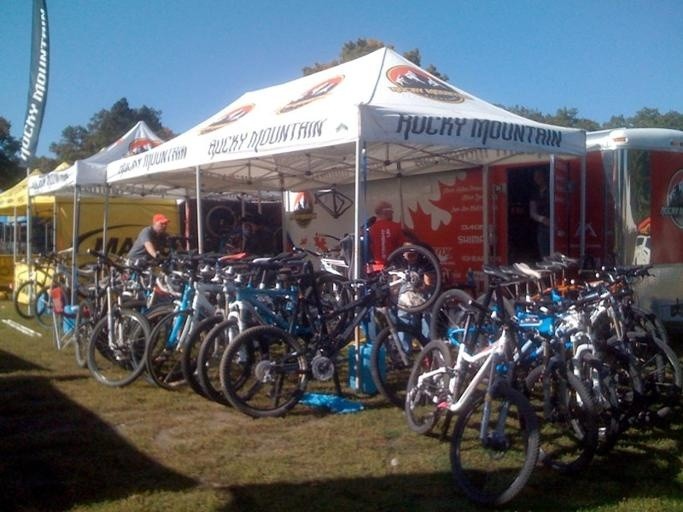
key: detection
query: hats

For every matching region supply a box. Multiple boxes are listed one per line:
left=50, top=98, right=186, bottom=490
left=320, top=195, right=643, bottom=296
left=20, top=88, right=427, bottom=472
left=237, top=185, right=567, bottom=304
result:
left=153, top=214, right=170, bottom=224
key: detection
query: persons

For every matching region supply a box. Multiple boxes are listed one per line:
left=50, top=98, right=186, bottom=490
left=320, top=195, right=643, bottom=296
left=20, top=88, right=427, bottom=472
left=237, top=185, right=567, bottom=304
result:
left=528, top=167, right=551, bottom=259
left=212, top=214, right=273, bottom=257
left=121, top=214, right=180, bottom=286
left=508, top=168, right=536, bottom=267
left=325, top=199, right=416, bottom=275
